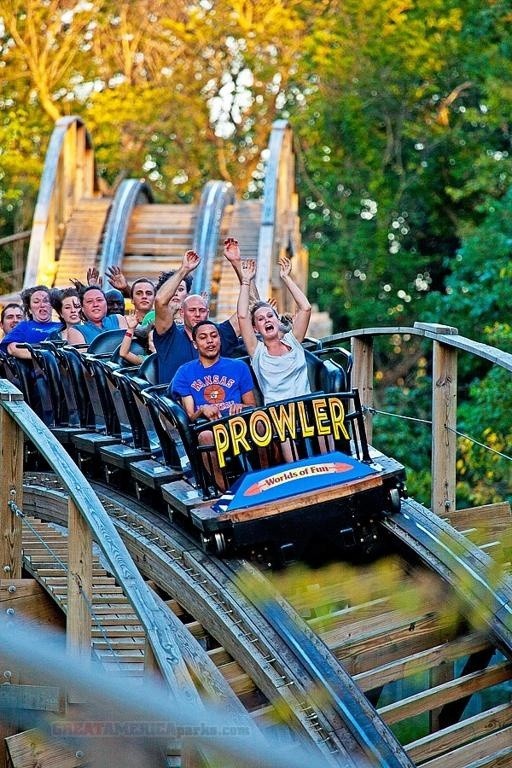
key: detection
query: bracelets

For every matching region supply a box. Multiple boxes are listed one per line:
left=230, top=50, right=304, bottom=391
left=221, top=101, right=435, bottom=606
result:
left=240, top=280, right=250, bottom=286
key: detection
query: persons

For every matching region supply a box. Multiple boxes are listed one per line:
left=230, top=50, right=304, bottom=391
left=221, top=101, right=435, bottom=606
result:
left=237, top=311, right=293, bottom=356
left=237, top=258, right=336, bottom=464
left=168, top=321, right=281, bottom=493
left=153, top=236, right=261, bottom=385
left=0, top=266, right=209, bottom=366
